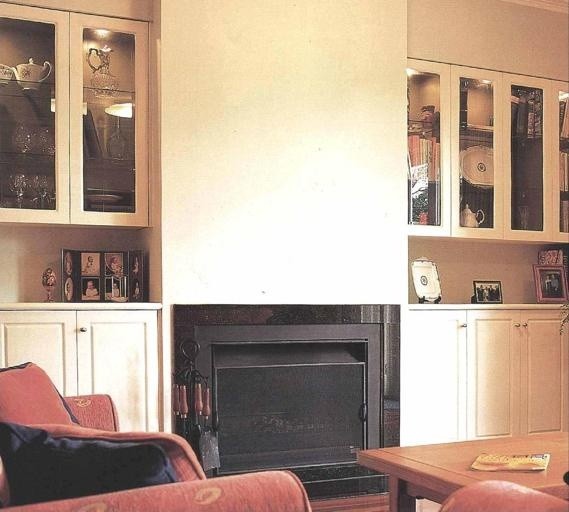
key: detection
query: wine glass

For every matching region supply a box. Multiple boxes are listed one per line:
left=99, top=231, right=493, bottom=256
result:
left=14, top=126, right=54, bottom=155
left=9, top=174, right=52, bottom=207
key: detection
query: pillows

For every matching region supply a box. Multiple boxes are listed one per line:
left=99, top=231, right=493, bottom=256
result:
left=26, top=423, right=206, bottom=482
left=0, top=421, right=178, bottom=505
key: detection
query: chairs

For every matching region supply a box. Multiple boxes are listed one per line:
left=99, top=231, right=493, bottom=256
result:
left=439, top=478, right=569, bottom=511
left=1, top=364, right=312, bottom=512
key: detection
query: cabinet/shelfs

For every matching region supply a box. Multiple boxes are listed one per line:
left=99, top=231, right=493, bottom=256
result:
left=410, top=310, right=569, bottom=443
left=1, top=1, right=149, bottom=230
left=0, top=308, right=160, bottom=433
left=406, top=58, right=569, bottom=242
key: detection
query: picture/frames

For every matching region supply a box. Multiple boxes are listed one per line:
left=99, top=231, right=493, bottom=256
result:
left=473, top=280, right=504, bottom=304
left=532, top=263, right=569, bottom=303
left=60, top=247, right=144, bottom=302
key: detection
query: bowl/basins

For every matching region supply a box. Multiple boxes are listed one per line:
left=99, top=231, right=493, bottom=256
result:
left=0, top=63, right=12, bottom=85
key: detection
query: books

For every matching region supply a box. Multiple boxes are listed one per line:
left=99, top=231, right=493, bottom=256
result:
left=408, top=128, right=440, bottom=226
left=471, top=453, right=552, bottom=472
left=515, top=88, right=569, bottom=232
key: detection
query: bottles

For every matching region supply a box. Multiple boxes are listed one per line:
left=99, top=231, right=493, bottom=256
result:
left=519, top=192, right=531, bottom=229
left=109, top=114, right=126, bottom=162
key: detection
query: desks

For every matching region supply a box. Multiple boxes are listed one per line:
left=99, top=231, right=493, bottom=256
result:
left=357, top=433, right=569, bottom=512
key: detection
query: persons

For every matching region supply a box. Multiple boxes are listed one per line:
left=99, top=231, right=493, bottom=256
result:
left=110, top=256, right=121, bottom=270
left=550, top=275, right=559, bottom=294
left=544, top=274, right=551, bottom=295
left=66, top=282, right=72, bottom=298
left=132, top=256, right=140, bottom=273
left=477, top=283, right=500, bottom=301
left=133, top=282, right=140, bottom=295
left=85, top=279, right=98, bottom=297
left=65, top=255, right=72, bottom=274
left=112, top=283, right=119, bottom=296
left=84, top=255, right=98, bottom=275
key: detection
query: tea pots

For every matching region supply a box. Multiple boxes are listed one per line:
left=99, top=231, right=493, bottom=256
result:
left=461, top=203, right=484, bottom=227
left=12, top=58, right=52, bottom=90
left=87, top=46, right=119, bottom=98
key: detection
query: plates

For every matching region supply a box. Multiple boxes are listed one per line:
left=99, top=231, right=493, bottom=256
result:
left=459, top=145, right=495, bottom=191
left=85, top=188, right=127, bottom=204
